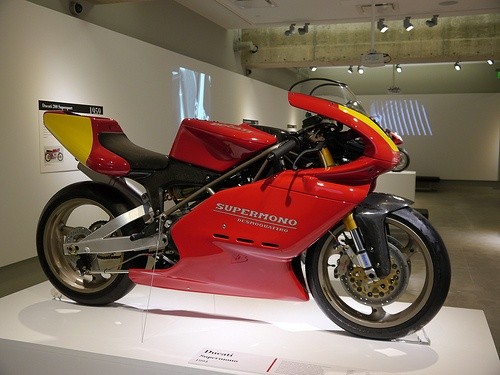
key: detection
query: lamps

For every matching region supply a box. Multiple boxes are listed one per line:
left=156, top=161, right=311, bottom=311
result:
left=309, top=67, right=317, bottom=71
left=377, top=14, right=438, bottom=33
left=487, top=59, right=494, bottom=65
left=348, top=65, right=363, bottom=74
left=396, top=64, right=402, bottom=72
left=284, top=23, right=309, bottom=36
left=454, top=62, right=461, bottom=71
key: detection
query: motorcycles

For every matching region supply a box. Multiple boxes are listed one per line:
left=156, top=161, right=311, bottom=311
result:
left=385, top=129, right=410, bottom=172
left=35, top=78, right=452, bottom=342
